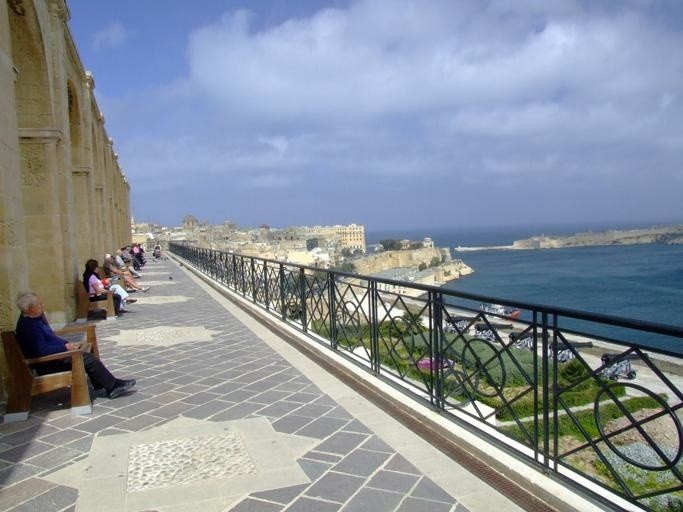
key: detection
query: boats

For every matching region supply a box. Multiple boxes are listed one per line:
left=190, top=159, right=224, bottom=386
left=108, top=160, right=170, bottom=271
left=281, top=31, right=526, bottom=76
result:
left=479, top=303, right=521, bottom=319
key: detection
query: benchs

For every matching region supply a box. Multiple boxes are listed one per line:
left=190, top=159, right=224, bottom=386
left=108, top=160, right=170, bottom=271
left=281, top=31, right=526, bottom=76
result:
left=1, top=324, right=99, bottom=424
left=75, top=254, right=134, bottom=324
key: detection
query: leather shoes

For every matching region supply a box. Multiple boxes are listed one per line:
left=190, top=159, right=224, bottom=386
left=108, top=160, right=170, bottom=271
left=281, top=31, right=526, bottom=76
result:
left=94, top=378, right=136, bottom=399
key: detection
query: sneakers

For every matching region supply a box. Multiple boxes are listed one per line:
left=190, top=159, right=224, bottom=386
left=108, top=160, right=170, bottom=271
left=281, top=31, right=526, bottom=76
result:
left=143, top=286, right=151, bottom=292
left=126, top=287, right=135, bottom=293
left=125, top=298, right=137, bottom=305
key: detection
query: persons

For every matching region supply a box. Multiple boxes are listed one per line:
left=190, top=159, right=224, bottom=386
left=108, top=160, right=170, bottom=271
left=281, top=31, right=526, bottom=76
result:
left=82, top=240, right=151, bottom=318
left=153, top=241, right=161, bottom=258
left=16, top=291, right=136, bottom=399
